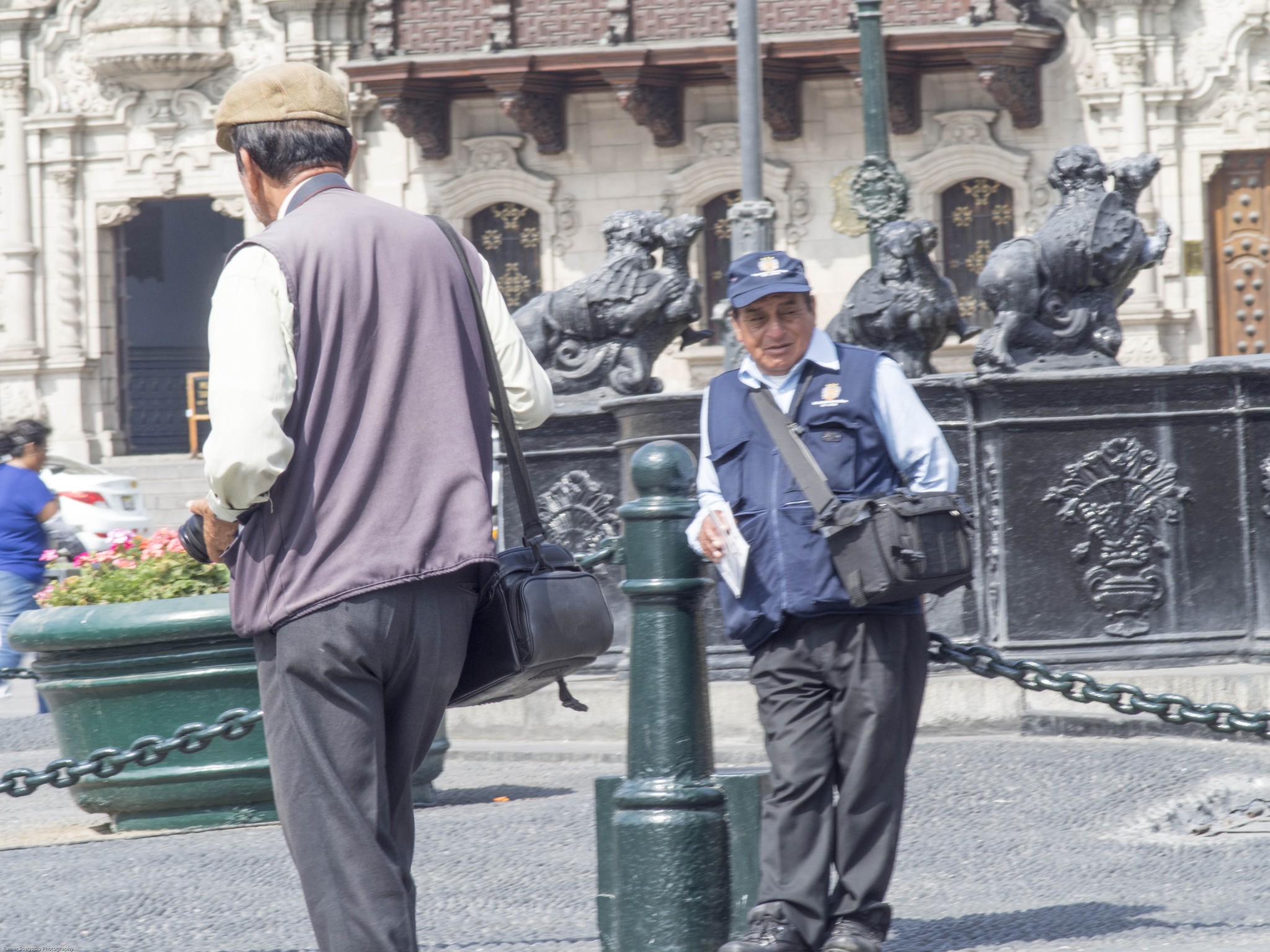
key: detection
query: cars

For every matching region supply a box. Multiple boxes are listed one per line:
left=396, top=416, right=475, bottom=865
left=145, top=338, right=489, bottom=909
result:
left=0, top=454, right=150, bottom=563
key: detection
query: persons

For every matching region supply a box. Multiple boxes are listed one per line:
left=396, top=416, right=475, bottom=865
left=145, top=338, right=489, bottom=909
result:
left=0, top=417, right=90, bottom=715
left=185, top=62, right=555, bottom=952
left=685, top=251, right=961, bottom=952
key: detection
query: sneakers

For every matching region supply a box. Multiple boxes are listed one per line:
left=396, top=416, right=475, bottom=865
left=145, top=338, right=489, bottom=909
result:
left=818, top=919, right=884, bottom=952
left=717, top=912, right=814, bottom=952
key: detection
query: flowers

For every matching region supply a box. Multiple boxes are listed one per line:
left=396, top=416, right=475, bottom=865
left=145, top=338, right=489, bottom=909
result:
left=22, top=526, right=232, bottom=605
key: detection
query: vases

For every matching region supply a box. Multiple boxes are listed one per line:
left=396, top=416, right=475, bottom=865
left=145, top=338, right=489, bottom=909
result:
left=6, top=588, right=450, bottom=835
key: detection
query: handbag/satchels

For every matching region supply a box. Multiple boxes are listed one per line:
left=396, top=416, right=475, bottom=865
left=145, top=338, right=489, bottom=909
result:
left=447, top=542, right=615, bottom=710
left=819, top=486, right=975, bottom=607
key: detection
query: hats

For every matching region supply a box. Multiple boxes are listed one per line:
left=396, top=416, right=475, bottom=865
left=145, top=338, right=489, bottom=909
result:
left=724, top=251, right=811, bottom=309
left=215, top=61, right=351, bottom=153
